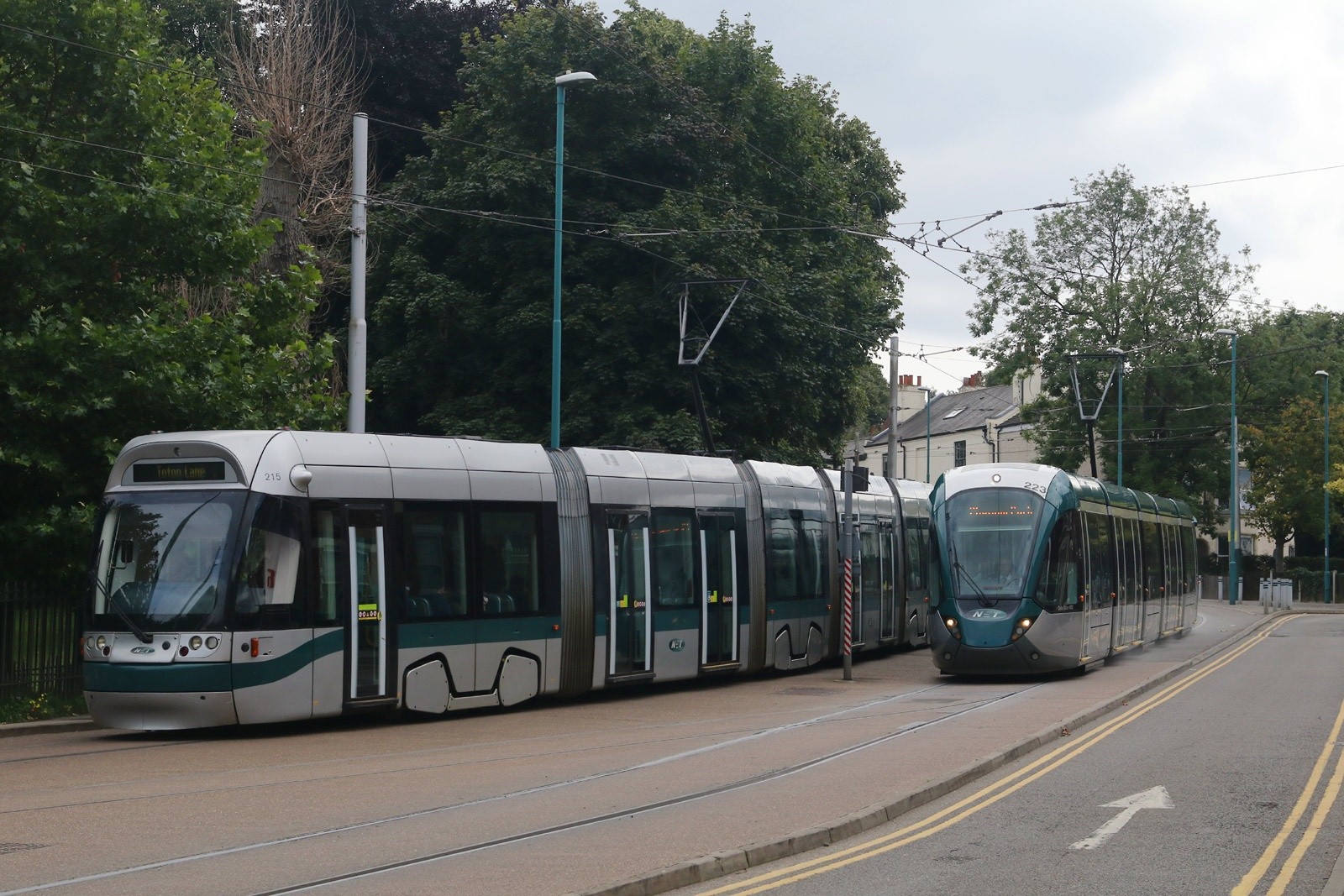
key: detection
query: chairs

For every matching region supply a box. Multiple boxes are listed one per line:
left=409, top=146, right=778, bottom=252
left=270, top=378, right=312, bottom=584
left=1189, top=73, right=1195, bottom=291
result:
left=406, top=593, right=517, bottom=618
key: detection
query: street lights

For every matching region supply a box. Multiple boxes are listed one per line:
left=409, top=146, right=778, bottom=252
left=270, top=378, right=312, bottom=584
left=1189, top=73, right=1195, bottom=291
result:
left=550, top=69, right=599, bottom=449
left=1215, top=328, right=1237, bottom=604
left=1106, top=346, right=1124, bottom=486
left=916, top=385, right=931, bottom=483
left=1314, top=370, right=1332, bottom=604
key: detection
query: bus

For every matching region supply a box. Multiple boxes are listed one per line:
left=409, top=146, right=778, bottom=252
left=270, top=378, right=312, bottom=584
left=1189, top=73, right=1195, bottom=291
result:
left=74, top=281, right=940, bottom=733
left=926, top=351, right=1201, bottom=681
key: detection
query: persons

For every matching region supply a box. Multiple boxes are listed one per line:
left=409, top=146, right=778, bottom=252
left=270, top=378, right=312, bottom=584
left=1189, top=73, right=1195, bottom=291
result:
left=990, top=558, right=1019, bottom=587
